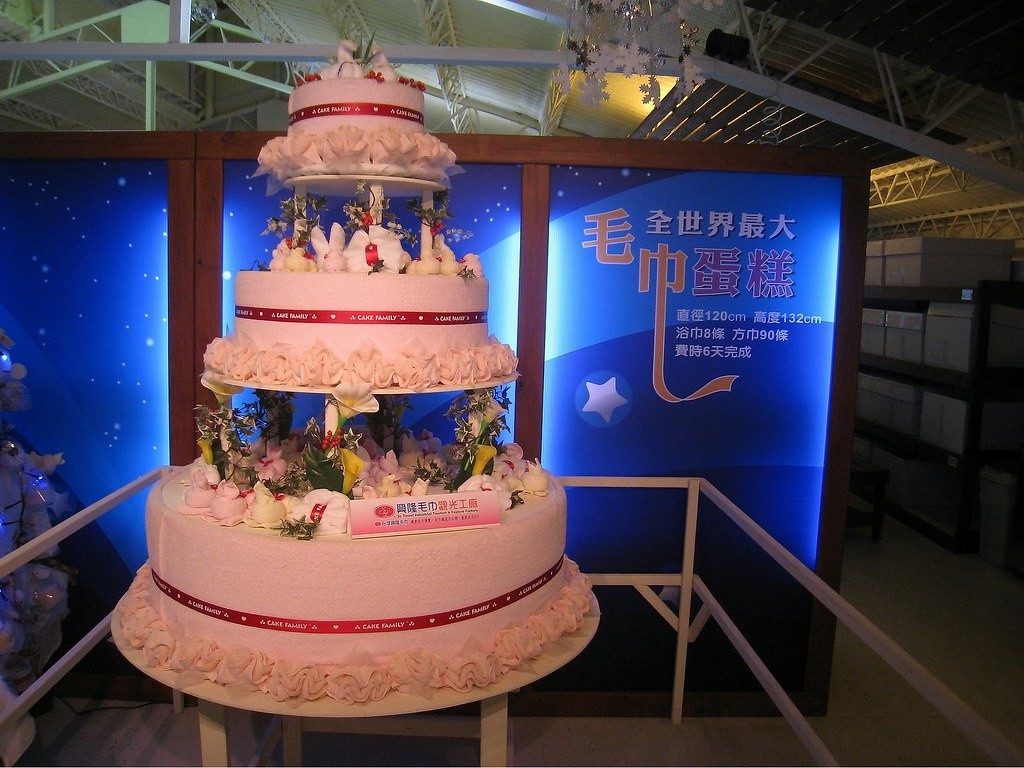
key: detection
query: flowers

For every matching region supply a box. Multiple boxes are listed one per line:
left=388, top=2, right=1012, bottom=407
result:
left=193, top=377, right=524, bottom=541
left=267, top=177, right=477, bottom=284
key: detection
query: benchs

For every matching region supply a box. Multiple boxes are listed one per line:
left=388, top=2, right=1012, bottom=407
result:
left=845, top=455, right=891, bottom=540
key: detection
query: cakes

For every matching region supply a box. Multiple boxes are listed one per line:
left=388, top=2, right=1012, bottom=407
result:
left=202, top=221, right=519, bottom=392
left=257, top=40, right=457, bottom=183
left=115, top=421, right=593, bottom=703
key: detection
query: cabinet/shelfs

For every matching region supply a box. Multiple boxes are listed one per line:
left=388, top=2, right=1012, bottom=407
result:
left=852, top=258, right=1024, bottom=555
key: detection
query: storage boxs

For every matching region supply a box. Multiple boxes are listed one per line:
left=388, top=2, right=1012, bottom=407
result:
left=856, top=372, right=1024, bottom=455
left=860, top=302, right=1024, bottom=372
left=864, top=236, right=1024, bottom=286
left=853, top=436, right=959, bottom=508
